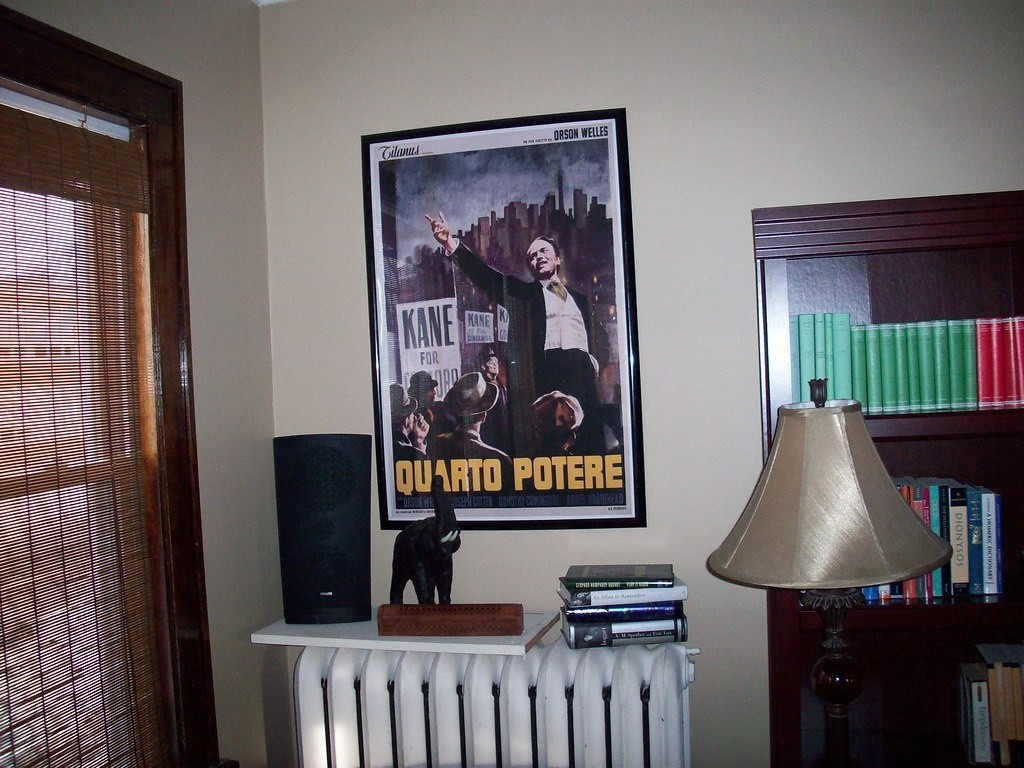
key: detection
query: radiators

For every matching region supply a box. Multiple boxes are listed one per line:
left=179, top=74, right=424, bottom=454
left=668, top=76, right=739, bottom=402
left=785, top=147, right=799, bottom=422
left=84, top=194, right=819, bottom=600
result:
left=293, top=645, right=703, bottom=768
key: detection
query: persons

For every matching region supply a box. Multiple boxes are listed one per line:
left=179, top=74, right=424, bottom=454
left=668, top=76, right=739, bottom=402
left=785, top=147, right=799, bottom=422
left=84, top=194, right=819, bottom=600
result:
left=388, top=347, right=514, bottom=461
left=518, top=390, right=585, bottom=459
left=425, top=211, right=608, bottom=456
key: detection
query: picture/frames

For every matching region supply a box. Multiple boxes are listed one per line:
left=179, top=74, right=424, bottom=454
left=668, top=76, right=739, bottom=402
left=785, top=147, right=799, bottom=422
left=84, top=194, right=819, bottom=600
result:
left=364, top=113, right=648, bottom=530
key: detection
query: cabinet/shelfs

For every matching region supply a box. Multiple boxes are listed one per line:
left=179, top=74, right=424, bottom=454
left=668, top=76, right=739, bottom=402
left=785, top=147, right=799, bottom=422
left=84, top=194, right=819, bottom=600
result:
left=750, top=190, right=1024, bottom=768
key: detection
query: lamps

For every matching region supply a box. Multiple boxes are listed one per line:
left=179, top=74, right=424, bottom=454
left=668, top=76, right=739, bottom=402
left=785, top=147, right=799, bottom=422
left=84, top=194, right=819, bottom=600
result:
left=706, top=377, right=953, bottom=768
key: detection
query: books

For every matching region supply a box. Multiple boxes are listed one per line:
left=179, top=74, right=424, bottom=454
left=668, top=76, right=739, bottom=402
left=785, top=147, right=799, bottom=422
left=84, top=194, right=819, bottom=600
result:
left=958, top=643, right=1024, bottom=766
left=555, top=564, right=688, bottom=649
left=789, top=312, right=1024, bottom=416
left=860, top=475, right=1004, bottom=600
